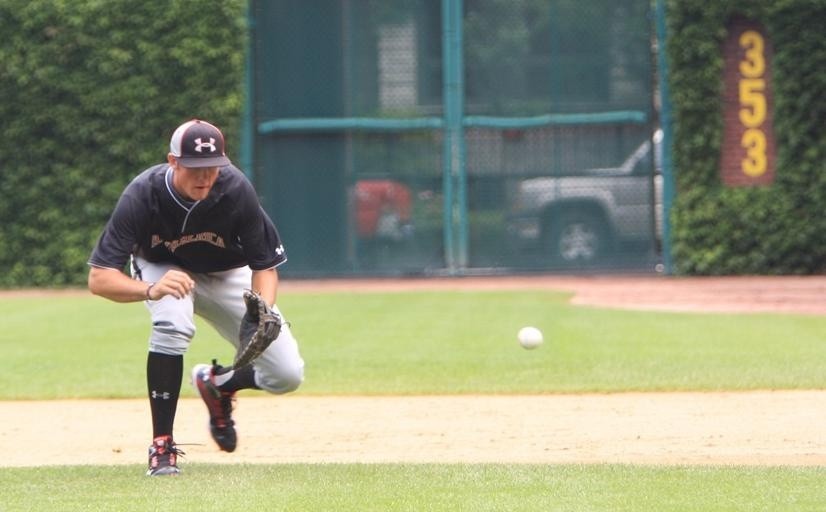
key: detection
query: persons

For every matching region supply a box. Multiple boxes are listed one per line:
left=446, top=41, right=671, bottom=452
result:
left=88, top=119, right=303, bottom=480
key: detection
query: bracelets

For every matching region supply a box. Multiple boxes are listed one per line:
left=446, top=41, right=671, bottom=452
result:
left=147, top=281, right=155, bottom=301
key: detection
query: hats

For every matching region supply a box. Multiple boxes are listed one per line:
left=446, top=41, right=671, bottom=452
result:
left=169, top=119, right=231, bottom=169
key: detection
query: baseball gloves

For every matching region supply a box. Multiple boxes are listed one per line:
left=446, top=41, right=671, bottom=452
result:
left=232, top=290, right=281, bottom=369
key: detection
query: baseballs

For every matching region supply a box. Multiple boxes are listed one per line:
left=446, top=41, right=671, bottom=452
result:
left=518, top=326, right=543, bottom=348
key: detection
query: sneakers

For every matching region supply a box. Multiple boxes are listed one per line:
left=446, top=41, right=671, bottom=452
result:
left=191, top=359, right=237, bottom=452
left=146, top=437, right=186, bottom=478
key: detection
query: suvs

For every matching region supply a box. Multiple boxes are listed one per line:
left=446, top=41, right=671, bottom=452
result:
left=509, top=128, right=667, bottom=269
